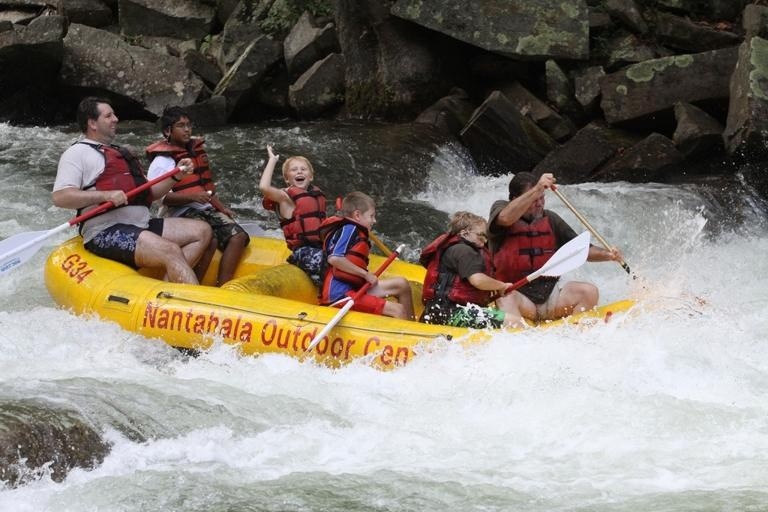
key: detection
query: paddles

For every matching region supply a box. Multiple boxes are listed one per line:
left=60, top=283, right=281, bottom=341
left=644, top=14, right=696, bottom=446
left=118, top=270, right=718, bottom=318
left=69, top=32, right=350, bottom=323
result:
left=0, top=166, right=186, bottom=275
left=298, top=242, right=407, bottom=359
left=486, top=230, right=590, bottom=303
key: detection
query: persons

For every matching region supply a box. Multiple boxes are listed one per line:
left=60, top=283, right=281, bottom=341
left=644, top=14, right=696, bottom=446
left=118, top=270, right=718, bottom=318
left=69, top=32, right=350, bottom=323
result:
left=487, top=171, right=624, bottom=329
left=51, top=95, right=212, bottom=286
left=419, top=210, right=528, bottom=331
left=258, top=143, right=415, bottom=320
left=146, top=103, right=251, bottom=286
left=321, top=191, right=407, bottom=319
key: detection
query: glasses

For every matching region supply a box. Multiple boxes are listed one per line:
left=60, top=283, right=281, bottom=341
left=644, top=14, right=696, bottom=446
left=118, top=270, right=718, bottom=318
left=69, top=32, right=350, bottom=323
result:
left=469, top=230, right=488, bottom=244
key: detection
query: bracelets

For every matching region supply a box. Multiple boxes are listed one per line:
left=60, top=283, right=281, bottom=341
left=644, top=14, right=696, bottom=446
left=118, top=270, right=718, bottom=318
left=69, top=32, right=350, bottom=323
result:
left=169, top=173, right=182, bottom=183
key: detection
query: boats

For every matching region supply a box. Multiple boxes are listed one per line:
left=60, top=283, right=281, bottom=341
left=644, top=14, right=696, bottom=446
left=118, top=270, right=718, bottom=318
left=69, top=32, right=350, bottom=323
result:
left=41, top=223, right=648, bottom=374
